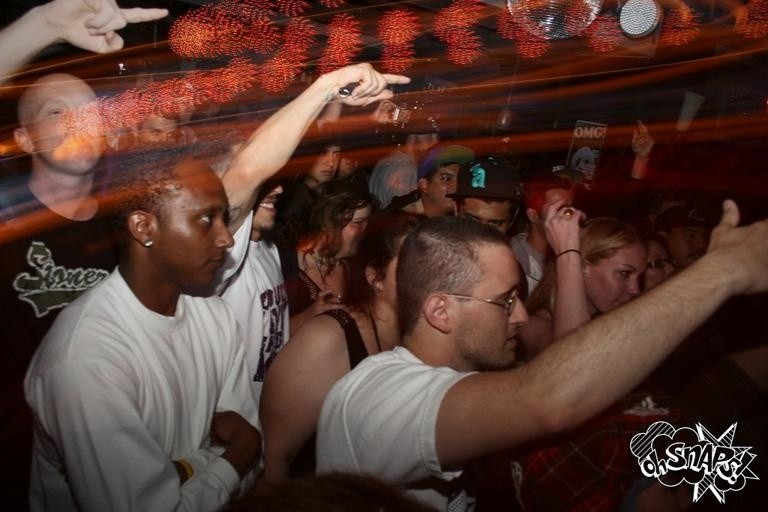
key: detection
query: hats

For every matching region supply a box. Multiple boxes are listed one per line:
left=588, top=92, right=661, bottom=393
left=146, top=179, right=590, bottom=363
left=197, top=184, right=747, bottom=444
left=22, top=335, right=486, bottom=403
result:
left=445, top=157, right=521, bottom=200
left=417, top=145, right=476, bottom=181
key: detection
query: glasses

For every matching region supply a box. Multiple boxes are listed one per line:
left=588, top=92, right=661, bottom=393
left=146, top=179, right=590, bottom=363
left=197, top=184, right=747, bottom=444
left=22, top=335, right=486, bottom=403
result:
left=446, top=294, right=517, bottom=316
left=646, top=258, right=668, bottom=269
